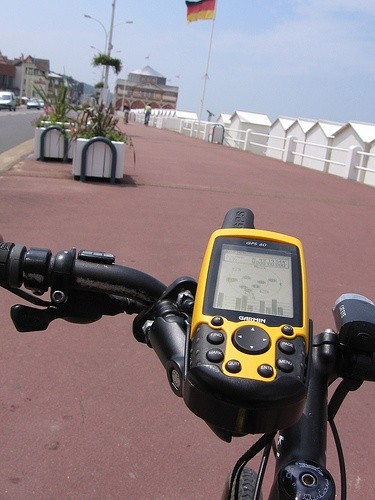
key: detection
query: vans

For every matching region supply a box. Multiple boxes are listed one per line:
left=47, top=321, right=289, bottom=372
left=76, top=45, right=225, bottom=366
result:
left=0, top=91, right=17, bottom=111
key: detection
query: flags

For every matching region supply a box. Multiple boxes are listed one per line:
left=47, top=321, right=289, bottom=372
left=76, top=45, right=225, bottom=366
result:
left=185, top=0, right=215, bottom=21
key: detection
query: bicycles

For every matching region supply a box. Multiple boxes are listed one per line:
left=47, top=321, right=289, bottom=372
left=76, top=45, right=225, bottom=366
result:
left=0, top=207, right=375, bottom=500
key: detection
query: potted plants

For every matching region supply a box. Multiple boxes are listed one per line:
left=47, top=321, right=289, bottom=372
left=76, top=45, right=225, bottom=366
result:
left=90, top=53, right=123, bottom=77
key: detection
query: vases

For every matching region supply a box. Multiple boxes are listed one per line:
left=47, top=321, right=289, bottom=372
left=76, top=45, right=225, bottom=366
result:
left=34, top=121, right=75, bottom=164
left=71, top=136, right=126, bottom=185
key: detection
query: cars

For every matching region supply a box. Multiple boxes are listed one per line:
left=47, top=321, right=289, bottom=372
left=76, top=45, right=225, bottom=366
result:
left=27, top=98, right=41, bottom=110
left=39, top=99, right=44, bottom=108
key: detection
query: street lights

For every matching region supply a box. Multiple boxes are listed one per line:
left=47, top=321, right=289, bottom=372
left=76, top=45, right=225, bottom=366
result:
left=90, top=45, right=122, bottom=95
left=84, top=14, right=136, bottom=111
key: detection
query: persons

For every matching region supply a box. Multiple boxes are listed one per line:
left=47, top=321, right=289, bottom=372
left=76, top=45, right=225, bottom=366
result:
left=143, top=104, right=152, bottom=124
left=123, top=103, right=130, bottom=123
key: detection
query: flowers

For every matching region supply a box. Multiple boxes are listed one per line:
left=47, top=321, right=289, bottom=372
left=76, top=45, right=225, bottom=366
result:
left=27, top=76, right=138, bottom=185
